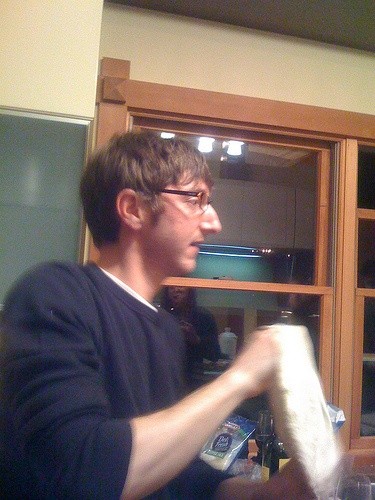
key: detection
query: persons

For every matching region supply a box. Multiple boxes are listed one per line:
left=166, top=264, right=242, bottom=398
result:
left=0, top=128, right=343, bottom=500
left=159, top=285, right=222, bottom=388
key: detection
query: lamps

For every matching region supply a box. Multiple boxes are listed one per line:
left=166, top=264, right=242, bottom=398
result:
left=161, top=132, right=176, bottom=139
left=197, top=137, right=215, bottom=152
left=222, top=140, right=245, bottom=155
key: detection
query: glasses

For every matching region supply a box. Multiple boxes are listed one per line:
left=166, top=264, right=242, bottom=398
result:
left=156, top=186, right=209, bottom=210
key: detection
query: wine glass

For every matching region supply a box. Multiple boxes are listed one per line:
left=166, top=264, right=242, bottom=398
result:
left=255, top=409, right=274, bottom=467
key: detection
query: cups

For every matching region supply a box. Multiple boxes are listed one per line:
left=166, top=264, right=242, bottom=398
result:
left=334, top=472, right=372, bottom=500
